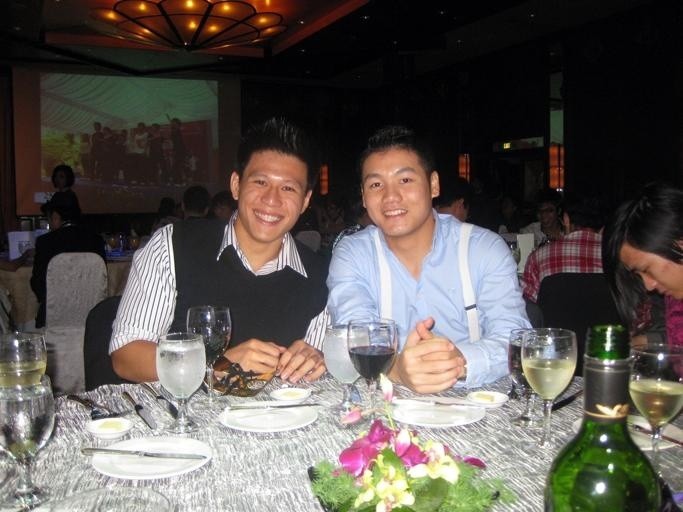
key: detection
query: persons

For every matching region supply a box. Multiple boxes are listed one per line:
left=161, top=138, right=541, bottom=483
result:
left=326, top=126, right=532, bottom=394
left=67, top=117, right=185, bottom=184
left=433, top=176, right=683, bottom=382
left=1, top=165, right=238, bottom=335
left=108, top=115, right=332, bottom=382
left=288, top=193, right=378, bottom=257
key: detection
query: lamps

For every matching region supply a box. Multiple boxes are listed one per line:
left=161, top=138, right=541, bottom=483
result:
left=65, top=0, right=300, bottom=57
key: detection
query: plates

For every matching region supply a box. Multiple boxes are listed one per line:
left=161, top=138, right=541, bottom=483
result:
left=270, top=386, right=310, bottom=401
left=82, top=416, right=133, bottom=440
left=43, top=486, right=177, bottom=512
left=219, top=402, right=318, bottom=433
left=92, top=437, right=210, bottom=480
left=625, top=415, right=682, bottom=454
left=390, top=396, right=486, bottom=428
left=466, top=390, right=509, bottom=409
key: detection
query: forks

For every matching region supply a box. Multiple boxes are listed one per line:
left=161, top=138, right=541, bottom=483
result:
left=64, top=393, right=120, bottom=419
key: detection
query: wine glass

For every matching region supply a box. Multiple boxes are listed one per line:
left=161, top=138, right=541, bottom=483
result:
left=18, top=241, right=30, bottom=254
left=626, top=343, right=681, bottom=480
left=0, top=332, right=46, bottom=388
left=154, top=331, right=206, bottom=434
left=349, top=315, right=399, bottom=410
left=185, top=303, right=231, bottom=410
left=0, top=372, right=54, bottom=508
left=322, top=322, right=368, bottom=416
left=520, top=328, right=578, bottom=453
left=506, top=324, right=548, bottom=430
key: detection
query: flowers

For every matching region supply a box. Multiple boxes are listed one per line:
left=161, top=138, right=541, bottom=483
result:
left=309, top=372, right=504, bottom=512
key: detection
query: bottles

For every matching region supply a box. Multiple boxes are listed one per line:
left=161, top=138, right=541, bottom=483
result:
left=540, top=326, right=669, bottom=512
left=103, top=232, right=138, bottom=255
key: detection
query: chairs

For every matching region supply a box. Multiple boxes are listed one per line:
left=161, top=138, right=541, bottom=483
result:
left=537, top=273, right=631, bottom=374
left=16, top=250, right=110, bottom=398
left=84, top=295, right=135, bottom=391
left=521, top=294, right=544, bottom=329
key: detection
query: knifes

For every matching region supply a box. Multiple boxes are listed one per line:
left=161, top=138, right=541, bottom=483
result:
left=628, top=417, right=681, bottom=447
left=396, top=395, right=474, bottom=410
left=79, top=446, right=205, bottom=463
left=139, top=382, right=178, bottom=418
left=225, top=403, right=320, bottom=412
left=548, top=390, right=586, bottom=412
left=121, top=391, right=157, bottom=431
